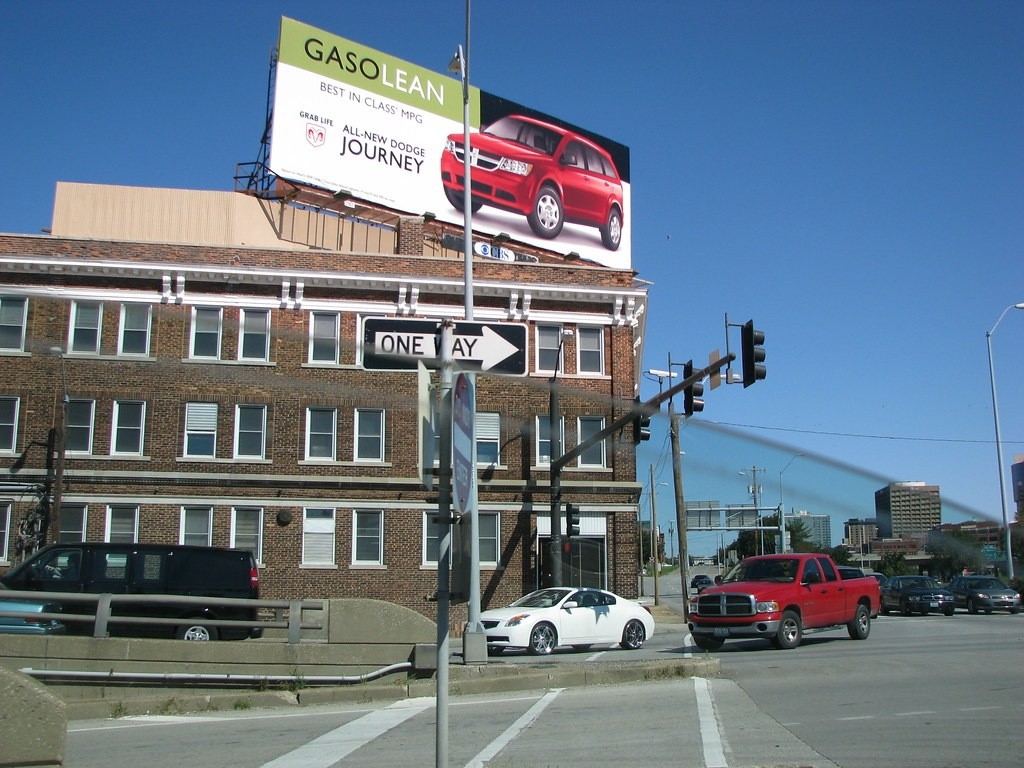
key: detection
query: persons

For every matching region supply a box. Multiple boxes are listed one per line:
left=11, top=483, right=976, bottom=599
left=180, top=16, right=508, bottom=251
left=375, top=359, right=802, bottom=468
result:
left=44, top=553, right=79, bottom=579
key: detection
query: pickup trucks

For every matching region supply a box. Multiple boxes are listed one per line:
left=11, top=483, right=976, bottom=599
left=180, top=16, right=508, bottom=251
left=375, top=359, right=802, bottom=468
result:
left=683, top=552, right=884, bottom=651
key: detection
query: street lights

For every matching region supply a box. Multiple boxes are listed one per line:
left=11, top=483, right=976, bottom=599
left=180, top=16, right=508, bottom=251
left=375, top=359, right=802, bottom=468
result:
left=647, top=481, right=668, bottom=578
left=778, top=452, right=806, bottom=505
left=546, top=330, right=576, bottom=589
left=49, top=344, right=71, bottom=546
left=984, top=303, right=1024, bottom=583
left=447, top=44, right=489, bottom=665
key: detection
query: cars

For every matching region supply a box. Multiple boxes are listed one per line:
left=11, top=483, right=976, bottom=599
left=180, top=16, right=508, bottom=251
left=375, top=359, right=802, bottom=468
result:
left=443, top=112, right=624, bottom=253
left=482, top=585, right=655, bottom=655
left=690, top=574, right=715, bottom=588
left=879, top=575, right=948, bottom=616
left=865, top=571, right=888, bottom=589
left=943, top=575, right=1021, bottom=614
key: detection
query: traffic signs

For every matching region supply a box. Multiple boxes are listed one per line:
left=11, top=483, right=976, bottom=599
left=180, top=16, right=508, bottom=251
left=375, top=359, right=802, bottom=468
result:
left=360, top=316, right=531, bottom=380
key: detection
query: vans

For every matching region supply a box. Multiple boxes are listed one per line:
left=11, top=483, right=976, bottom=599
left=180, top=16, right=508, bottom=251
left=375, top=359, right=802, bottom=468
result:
left=0, top=543, right=266, bottom=644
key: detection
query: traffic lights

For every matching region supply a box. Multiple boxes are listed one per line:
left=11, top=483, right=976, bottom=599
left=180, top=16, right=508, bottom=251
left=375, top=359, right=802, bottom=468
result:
left=682, top=359, right=704, bottom=418
left=633, top=395, right=650, bottom=446
left=741, top=320, right=767, bottom=387
left=566, top=502, right=579, bottom=538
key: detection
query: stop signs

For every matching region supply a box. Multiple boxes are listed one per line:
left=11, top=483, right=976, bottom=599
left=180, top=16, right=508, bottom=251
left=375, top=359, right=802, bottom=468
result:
left=449, top=369, right=476, bottom=517
